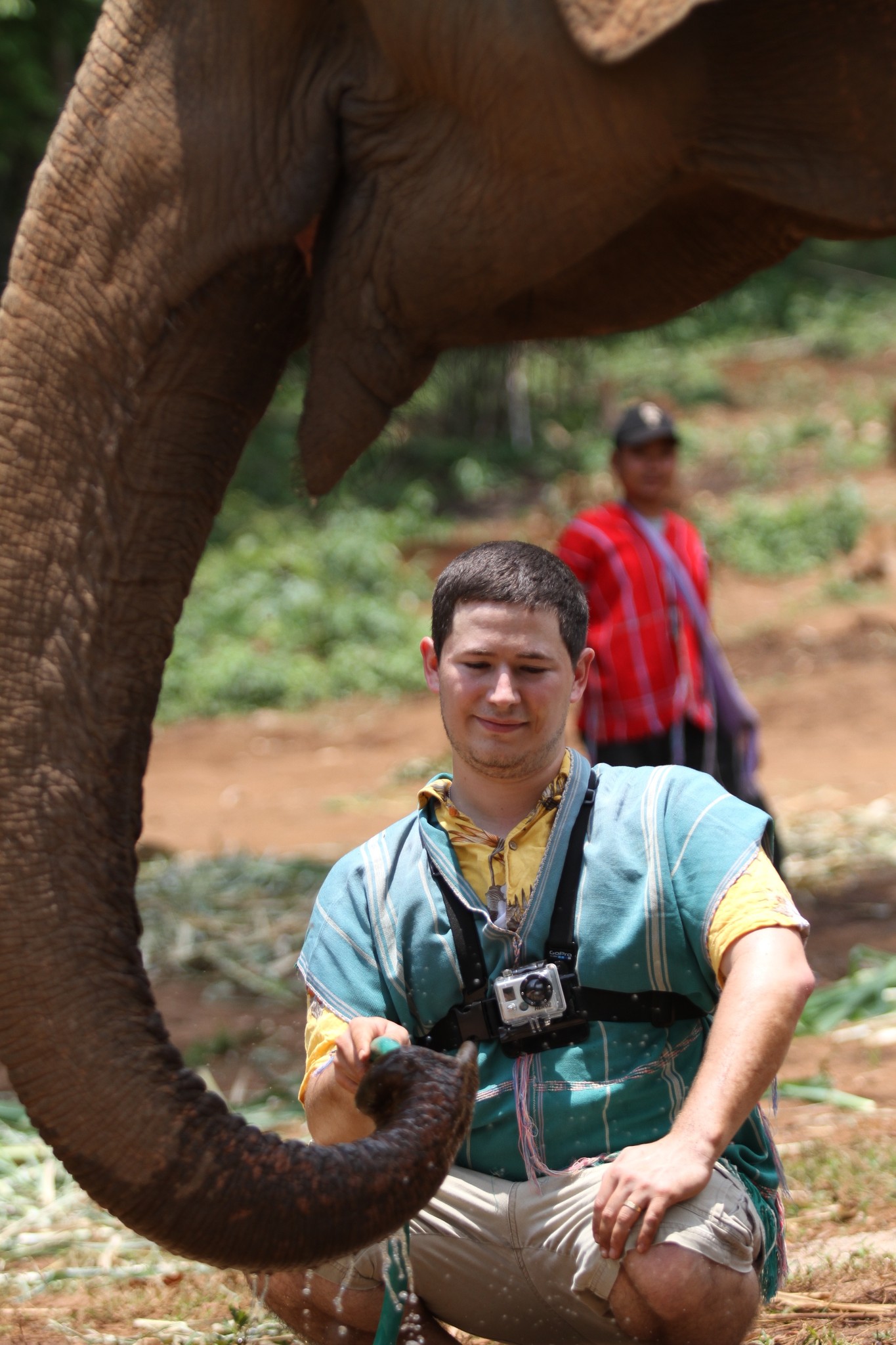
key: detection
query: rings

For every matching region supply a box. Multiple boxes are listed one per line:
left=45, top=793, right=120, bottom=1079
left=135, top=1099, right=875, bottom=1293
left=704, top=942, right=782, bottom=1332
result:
left=623, top=1199, right=643, bottom=1213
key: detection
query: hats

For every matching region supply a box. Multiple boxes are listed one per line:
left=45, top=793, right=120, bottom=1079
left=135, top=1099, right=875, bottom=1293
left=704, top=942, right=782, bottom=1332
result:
left=614, top=404, right=674, bottom=448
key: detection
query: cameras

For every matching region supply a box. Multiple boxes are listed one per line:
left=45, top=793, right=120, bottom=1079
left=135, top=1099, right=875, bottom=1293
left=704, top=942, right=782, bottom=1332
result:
left=488, top=958, right=591, bottom=1062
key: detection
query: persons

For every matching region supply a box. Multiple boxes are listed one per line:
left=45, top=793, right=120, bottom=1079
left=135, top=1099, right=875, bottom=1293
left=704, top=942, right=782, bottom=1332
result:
left=256, top=538, right=817, bottom=1345
left=553, top=401, right=740, bottom=794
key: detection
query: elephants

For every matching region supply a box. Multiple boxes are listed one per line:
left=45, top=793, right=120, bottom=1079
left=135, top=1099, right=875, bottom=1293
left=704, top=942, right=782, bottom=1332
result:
left=2, top=0, right=895, bottom=1271
